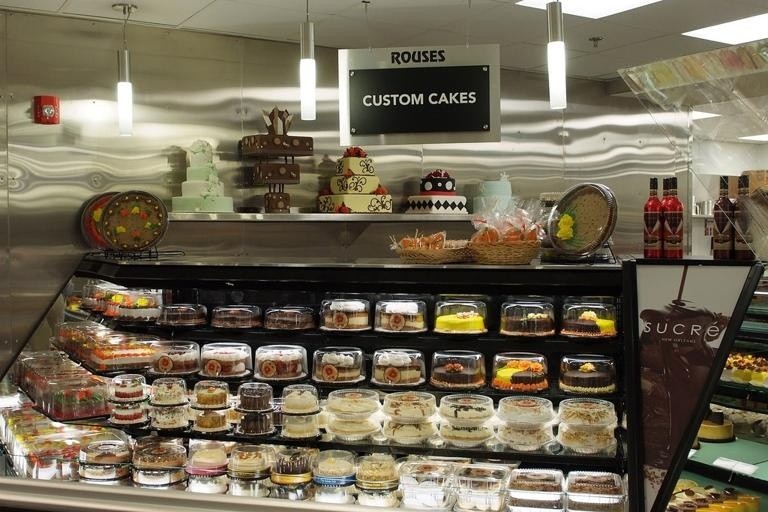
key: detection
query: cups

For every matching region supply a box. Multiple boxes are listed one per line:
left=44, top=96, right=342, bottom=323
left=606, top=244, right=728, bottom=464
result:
left=701, top=200, right=713, bottom=215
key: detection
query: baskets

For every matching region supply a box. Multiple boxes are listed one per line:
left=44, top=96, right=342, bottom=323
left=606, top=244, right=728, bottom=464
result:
left=466, top=240, right=541, bottom=265
left=394, top=239, right=468, bottom=265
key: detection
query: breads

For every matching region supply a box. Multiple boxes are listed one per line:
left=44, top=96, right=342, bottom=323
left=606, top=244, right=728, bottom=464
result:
left=399, top=233, right=445, bottom=251
left=473, top=225, right=540, bottom=243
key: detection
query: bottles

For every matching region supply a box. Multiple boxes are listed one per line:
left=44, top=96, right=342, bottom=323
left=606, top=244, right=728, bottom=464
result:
left=713, top=174, right=754, bottom=260
left=642, top=176, right=686, bottom=259
left=692, top=195, right=700, bottom=215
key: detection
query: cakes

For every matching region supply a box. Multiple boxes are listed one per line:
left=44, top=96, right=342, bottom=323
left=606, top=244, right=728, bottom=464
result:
left=697, top=352, right=768, bottom=442
left=1, top=405, right=623, bottom=510
left=240, top=106, right=314, bottom=213
left=14, top=351, right=620, bottom=453
left=68, top=279, right=620, bottom=340
left=320, top=147, right=391, bottom=213
left=473, top=170, right=513, bottom=213
left=666, top=479, right=759, bottom=512
left=403, top=170, right=469, bottom=214
left=52, top=317, right=616, bottom=396
left=171, top=139, right=235, bottom=214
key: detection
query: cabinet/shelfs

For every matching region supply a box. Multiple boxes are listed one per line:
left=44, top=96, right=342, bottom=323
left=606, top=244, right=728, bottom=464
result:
left=640, top=259, right=768, bottom=512
left=0, top=255, right=642, bottom=510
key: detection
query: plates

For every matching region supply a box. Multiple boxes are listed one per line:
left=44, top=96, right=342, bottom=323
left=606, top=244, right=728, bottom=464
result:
left=80, top=192, right=122, bottom=249
left=101, top=190, right=169, bottom=252
left=548, top=182, right=618, bottom=259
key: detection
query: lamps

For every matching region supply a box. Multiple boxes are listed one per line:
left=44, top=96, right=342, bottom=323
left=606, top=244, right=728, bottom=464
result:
left=112, top=2, right=141, bottom=143
left=298, top=0, right=320, bottom=122
left=544, top=1, right=569, bottom=112
